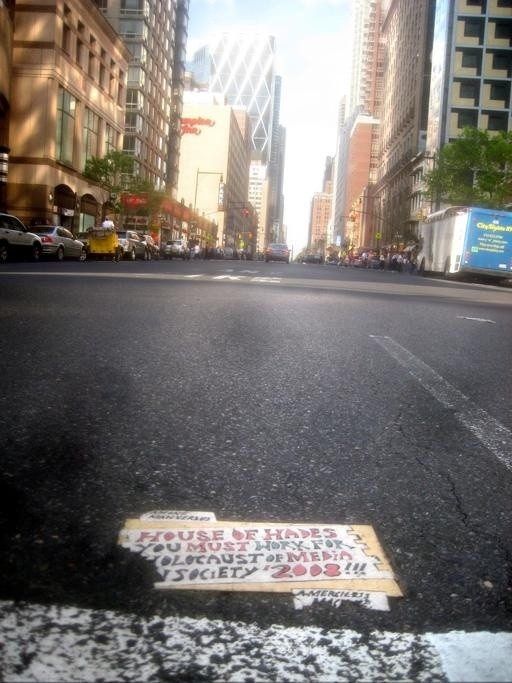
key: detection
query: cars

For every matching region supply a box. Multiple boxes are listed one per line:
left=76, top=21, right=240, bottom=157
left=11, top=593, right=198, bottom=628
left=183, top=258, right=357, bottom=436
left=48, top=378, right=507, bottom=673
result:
left=0, top=219, right=290, bottom=262
left=303, top=254, right=320, bottom=264
left=352, top=256, right=382, bottom=269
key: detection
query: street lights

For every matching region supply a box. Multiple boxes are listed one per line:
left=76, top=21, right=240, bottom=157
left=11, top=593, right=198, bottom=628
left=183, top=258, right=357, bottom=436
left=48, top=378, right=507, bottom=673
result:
left=189, top=168, right=224, bottom=213
left=360, top=195, right=381, bottom=247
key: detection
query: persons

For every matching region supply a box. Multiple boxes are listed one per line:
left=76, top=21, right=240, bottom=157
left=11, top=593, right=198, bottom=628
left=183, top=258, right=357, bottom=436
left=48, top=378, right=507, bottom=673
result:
left=181, top=234, right=254, bottom=260
left=346, top=245, right=415, bottom=271
left=102, top=215, right=115, bottom=229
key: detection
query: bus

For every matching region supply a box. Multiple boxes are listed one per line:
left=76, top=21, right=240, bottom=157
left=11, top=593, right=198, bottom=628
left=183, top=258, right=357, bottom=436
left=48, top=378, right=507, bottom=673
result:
left=412, top=206, right=512, bottom=285
